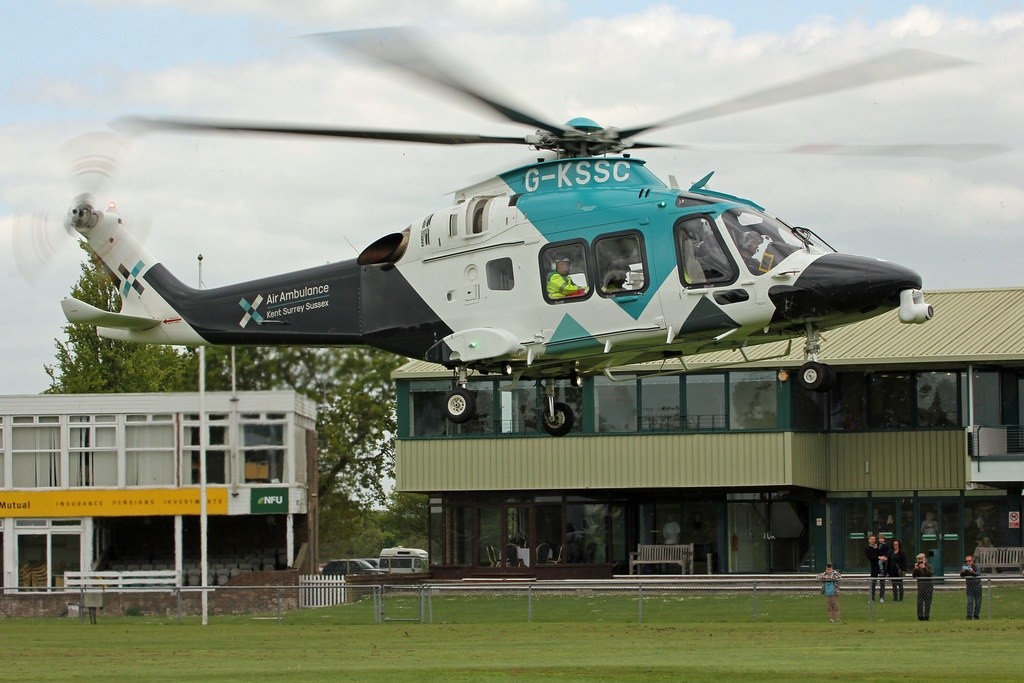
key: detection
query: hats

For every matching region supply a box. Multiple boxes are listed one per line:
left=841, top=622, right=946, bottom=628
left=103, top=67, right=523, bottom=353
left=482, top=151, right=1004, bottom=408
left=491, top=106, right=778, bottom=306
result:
left=916, top=553, right=926, bottom=558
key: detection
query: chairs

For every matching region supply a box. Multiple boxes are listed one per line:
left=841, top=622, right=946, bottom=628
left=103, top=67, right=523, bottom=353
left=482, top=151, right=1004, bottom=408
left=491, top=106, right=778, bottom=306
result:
left=105, top=557, right=276, bottom=586
left=486, top=541, right=599, bottom=567
left=17, top=562, right=48, bottom=592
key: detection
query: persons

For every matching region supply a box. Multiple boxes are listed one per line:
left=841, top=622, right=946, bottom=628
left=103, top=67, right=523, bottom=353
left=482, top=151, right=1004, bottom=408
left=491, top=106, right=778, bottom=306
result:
left=911, top=553, right=935, bottom=620
left=546, top=253, right=589, bottom=299
left=601, top=262, right=631, bottom=293
left=662, top=516, right=681, bottom=545
left=510, top=532, right=527, bottom=547
left=878, top=536, right=890, bottom=577
left=960, top=555, right=983, bottom=620
left=842, top=411, right=865, bottom=431
left=885, top=415, right=898, bottom=426
left=871, top=508, right=916, bottom=572
left=864, top=534, right=889, bottom=604
left=920, top=511, right=942, bottom=558
left=971, top=514, right=998, bottom=574
left=887, top=539, right=908, bottom=601
left=735, top=231, right=775, bottom=276
left=819, top=564, right=843, bottom=623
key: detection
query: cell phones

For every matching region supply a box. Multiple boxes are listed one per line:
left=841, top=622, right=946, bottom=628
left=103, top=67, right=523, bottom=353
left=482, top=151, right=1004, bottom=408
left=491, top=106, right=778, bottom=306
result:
left=917, top=562, right=923, bottom=565
left=964, top=566, right=971, bottom=570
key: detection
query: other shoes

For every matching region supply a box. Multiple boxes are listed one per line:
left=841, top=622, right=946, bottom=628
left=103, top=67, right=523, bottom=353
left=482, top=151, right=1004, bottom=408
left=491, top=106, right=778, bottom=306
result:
left=879, top=597, right=884, bottom=602
left=830, top=618, right=833, bottom=623
left=975, top=615, right=978, bottom=620
left=878, top=573, right=885, bottom=576
left=967, top=615, right=971, bottom=619
left=918, top=614, right=930, bottom=621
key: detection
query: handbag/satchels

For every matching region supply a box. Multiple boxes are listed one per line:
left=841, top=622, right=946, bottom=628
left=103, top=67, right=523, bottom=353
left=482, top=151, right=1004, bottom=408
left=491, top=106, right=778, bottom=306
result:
left=899, top=570, right=906, bottom=578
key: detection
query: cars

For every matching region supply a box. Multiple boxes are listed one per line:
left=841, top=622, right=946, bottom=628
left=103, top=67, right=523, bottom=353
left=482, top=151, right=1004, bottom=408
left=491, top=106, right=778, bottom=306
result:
left=317, top=557, right=380, bottom=593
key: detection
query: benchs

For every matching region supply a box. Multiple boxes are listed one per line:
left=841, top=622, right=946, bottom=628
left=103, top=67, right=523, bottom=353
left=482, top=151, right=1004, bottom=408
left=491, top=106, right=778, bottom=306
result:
left=973, top=546, right=1024, bottom=573
left=628, top=542, right=694, bottom=576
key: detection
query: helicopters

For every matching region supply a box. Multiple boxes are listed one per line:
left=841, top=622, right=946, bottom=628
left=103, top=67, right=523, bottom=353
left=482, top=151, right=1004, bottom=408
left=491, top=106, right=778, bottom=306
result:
left=20, top=17, right=972, bottom=438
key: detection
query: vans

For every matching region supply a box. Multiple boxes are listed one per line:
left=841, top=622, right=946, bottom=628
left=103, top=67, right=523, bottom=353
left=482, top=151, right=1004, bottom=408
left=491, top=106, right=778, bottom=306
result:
left=378, top=545, right=428, bottom=574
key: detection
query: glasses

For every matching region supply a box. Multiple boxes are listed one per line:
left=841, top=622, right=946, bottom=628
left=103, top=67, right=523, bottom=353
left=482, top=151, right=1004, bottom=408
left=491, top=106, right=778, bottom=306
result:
left=966, top=560, right=972, bottom=563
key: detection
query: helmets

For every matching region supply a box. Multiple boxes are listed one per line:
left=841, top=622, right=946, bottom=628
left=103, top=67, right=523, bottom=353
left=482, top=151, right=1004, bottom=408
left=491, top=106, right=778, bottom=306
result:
left=740, top=230, right=757, bottom=248
left=551, top=254, right=574, bottom=271
